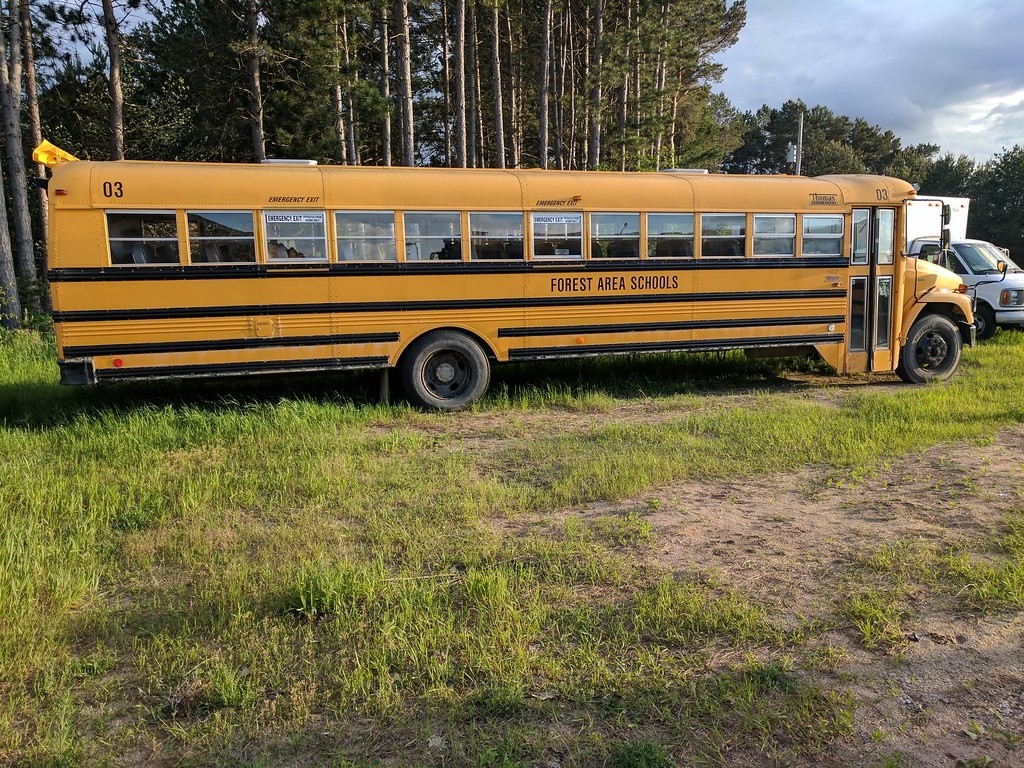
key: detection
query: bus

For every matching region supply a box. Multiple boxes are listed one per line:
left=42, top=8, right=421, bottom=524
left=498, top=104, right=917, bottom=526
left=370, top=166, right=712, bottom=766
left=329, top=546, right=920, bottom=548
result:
left=47, top=158, right=1008, bottom=413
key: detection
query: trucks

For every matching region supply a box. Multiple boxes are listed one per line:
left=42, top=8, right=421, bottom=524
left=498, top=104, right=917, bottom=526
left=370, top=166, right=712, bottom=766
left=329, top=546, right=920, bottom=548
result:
left=731, top=197, right=1024, bottom=343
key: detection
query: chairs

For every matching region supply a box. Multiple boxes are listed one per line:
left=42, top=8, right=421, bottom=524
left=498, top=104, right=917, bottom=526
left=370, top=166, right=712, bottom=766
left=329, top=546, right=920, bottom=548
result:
left=129, top=240, right=793, bottom=264
